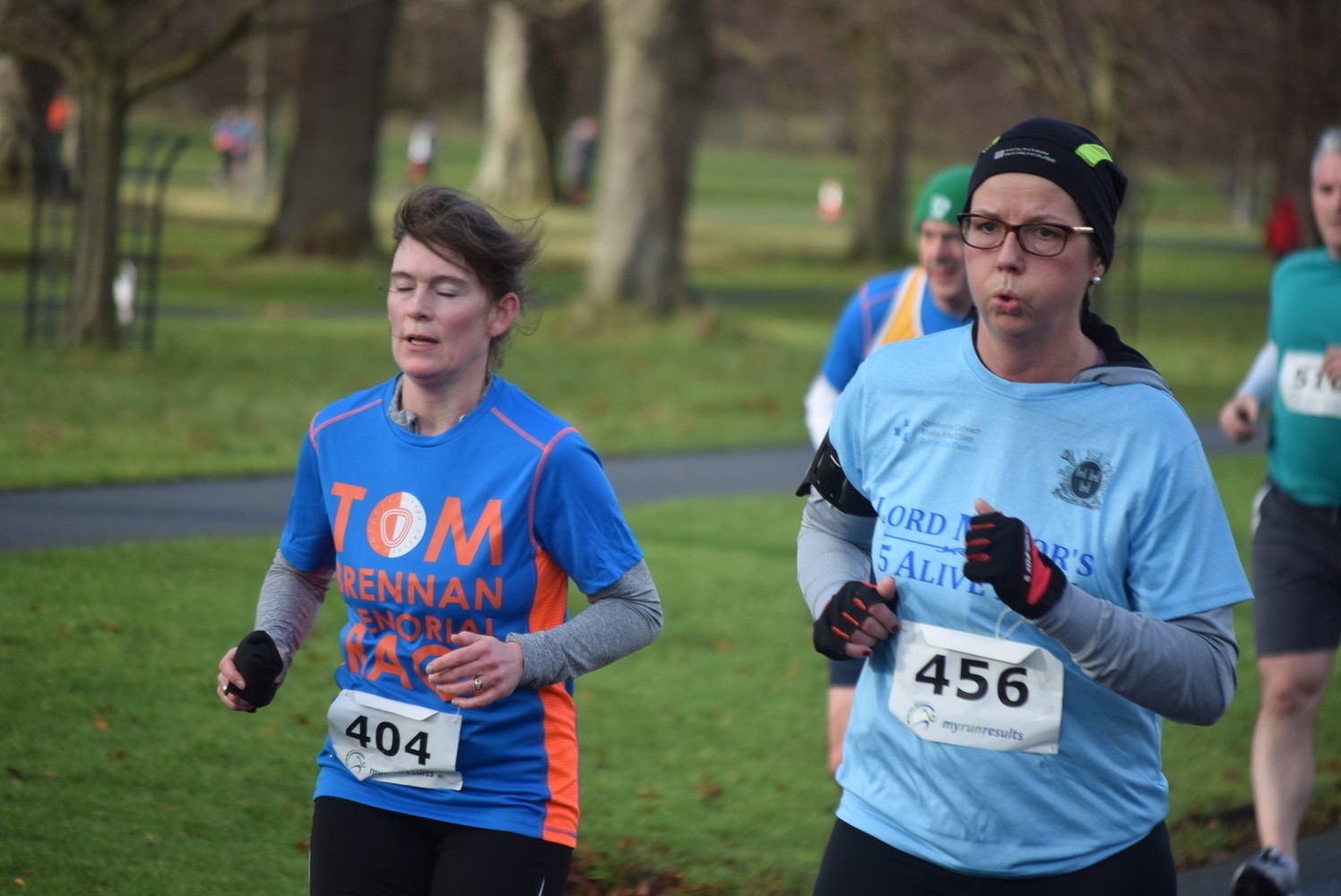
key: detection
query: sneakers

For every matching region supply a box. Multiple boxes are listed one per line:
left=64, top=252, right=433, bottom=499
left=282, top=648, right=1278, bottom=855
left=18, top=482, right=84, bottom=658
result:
left=1228, top=847, right=1300, bottom=895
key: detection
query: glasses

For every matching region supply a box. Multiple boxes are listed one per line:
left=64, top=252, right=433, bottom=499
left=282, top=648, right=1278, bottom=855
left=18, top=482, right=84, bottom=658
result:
left=957, top=213, right=1094, bottom=257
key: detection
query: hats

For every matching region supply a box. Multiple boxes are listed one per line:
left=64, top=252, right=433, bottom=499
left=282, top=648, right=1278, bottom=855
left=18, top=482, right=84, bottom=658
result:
left=971, top=117, right=1127, bottom=264
left=913, top=163, right=973, bottom=231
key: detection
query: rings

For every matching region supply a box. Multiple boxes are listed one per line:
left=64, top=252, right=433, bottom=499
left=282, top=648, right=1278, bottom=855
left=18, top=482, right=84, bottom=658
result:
left=222, top=685, right=230, bottom=696
left=473, top=676, right=482, bottom=691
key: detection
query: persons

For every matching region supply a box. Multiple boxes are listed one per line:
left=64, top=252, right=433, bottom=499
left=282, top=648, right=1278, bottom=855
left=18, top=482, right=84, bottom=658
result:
left=215, top=185, right=664, bottom=895
left=1217, top=128, right=1341, bottom=896
left=791, top=116, right=1258, bottom=895
